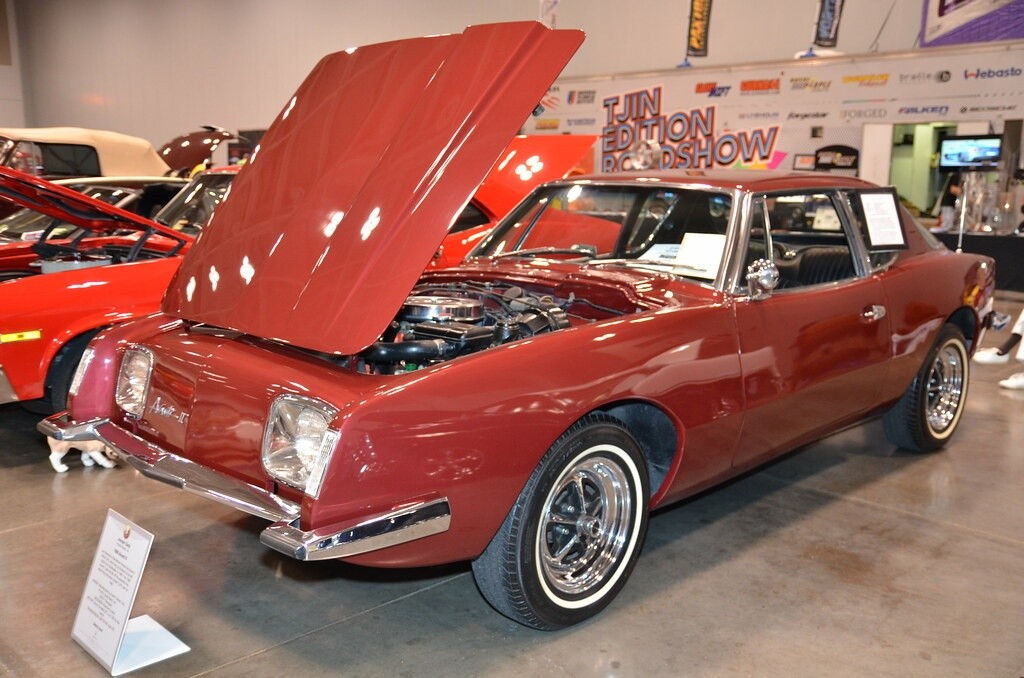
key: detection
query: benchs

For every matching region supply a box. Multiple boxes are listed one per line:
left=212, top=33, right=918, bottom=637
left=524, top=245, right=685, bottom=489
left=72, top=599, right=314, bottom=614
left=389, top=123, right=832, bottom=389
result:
left=741, top=238, right=853, bottom=287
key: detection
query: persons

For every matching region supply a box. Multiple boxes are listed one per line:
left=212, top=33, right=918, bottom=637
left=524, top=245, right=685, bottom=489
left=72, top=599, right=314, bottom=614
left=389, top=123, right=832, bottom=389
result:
left=938, top=171, right=966, bottom=231
left=972, top=301, right=1024, bottom=390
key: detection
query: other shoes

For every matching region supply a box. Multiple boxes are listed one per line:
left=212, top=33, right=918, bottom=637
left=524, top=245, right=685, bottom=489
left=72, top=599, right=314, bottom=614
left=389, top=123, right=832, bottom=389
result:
left=973, top=346, right=1009, bottom=364
left=999, top=372, right=1024, bottom=390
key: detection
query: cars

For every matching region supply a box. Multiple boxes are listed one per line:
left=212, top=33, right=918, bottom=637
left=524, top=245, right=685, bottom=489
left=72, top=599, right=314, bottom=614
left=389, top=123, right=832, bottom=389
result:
left=40, top=19, right=999, bottom=633
left=0, top=122, right=620, bottom=449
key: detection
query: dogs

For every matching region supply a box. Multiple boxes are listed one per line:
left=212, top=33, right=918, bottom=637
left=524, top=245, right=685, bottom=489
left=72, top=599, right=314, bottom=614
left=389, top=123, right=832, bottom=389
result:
left=46, top=434, right=120, bottom=474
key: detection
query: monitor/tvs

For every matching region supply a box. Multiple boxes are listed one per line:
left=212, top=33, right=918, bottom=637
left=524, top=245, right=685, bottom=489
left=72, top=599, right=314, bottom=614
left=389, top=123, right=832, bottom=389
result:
left=938, top=134, right=1003, bottom=172
